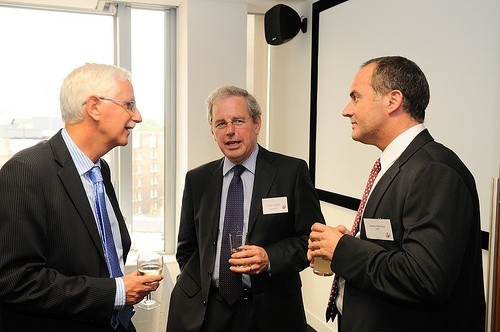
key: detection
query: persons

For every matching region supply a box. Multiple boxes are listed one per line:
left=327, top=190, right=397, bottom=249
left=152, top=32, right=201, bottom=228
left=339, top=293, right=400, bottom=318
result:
left=0, top=63, right=164, bottom=332
left=165, top=86, right=326, bottom=332
left=307, top=56, right=486, bottom=332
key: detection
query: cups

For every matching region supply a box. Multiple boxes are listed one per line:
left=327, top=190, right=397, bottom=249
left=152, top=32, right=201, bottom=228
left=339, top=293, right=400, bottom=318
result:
left=229, top=231, right=248, bottom=267
left=313, top=257, right=333, bottom=276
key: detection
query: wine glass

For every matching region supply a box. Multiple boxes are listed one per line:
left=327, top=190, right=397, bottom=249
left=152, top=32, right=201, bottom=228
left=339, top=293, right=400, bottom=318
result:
left=137, top=255, right=163, bottom=309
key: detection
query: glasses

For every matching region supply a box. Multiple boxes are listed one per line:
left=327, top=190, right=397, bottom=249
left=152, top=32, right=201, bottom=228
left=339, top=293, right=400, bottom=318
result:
left=210, top=115, right=253, bottom=129
left=82, top=97, right=136, bottom=112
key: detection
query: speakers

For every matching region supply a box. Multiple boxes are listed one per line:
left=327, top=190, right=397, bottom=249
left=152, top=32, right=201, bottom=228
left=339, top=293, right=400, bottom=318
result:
left=264, top=4, right=301, bottom=45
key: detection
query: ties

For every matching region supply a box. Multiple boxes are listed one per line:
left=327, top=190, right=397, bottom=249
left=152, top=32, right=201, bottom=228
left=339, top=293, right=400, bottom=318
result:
left=90, top=166, right=133, bottom=330
left=218, top=165, right=246, bottom=305
left=325, top=157, right=381, bottom=323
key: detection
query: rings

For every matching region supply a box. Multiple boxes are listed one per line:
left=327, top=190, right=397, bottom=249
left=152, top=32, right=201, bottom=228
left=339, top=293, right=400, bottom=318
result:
left=312, top=243, right=315, bottom=251
left=249, top=266, right=252, bottom=271
